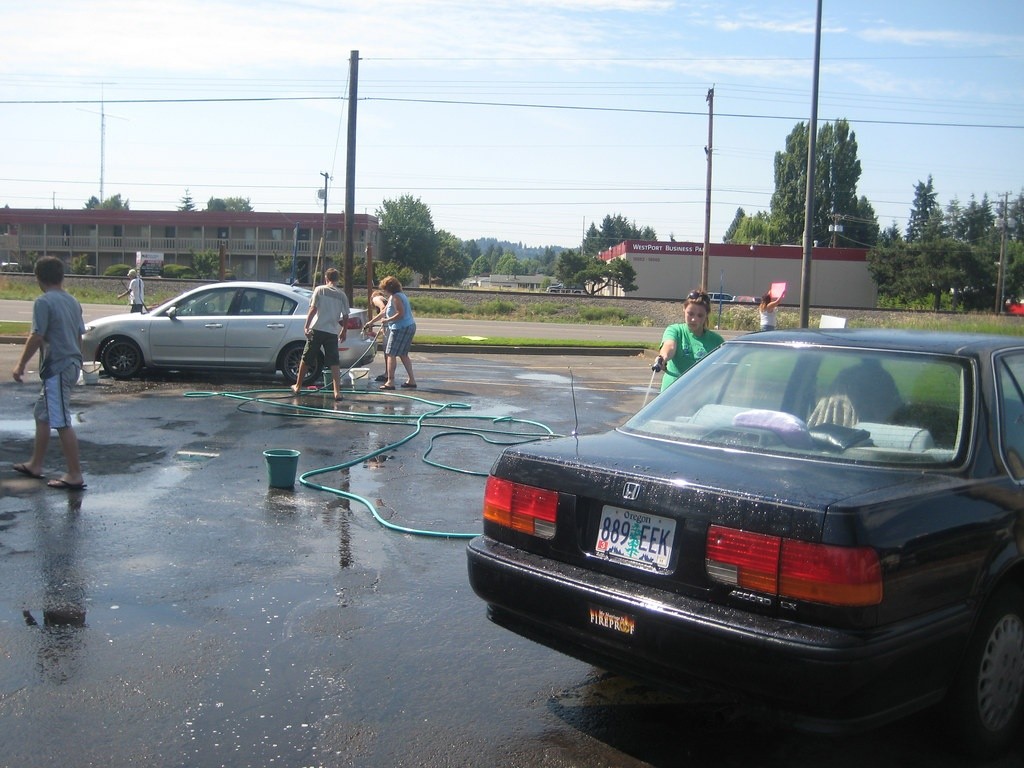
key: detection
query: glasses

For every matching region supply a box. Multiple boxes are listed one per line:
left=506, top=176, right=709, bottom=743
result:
left=687, top=292, right=710, bottom=302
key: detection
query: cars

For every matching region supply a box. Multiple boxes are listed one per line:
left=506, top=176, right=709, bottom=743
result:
left=547, top=283, right=585, bottom=294
left=71, top=281, right=376, bottom=386
left=466, top=329, right=1024, bottom=736
left=707, top=293, right=733, bottom=301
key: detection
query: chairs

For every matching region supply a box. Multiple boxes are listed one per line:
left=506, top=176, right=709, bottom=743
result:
left=240, top=294, right=258, bottom=315
left=690, top=404, right=935, bottom=450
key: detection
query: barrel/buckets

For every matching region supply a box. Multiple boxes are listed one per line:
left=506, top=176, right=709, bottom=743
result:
left=82, top=361, right=101, bottom=384
left=322, top=370, right=342, bottom=390
left=263, top=449, right=301, bottom=488
left=349, top=368, right=370, bottom=390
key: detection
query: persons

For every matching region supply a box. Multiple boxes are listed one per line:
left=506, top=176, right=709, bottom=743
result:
left=117, top=269, right=145, bottom=313
left=290, top=268, right=350, bottom=400
left=655, top=290, right=726, bottom=392
left=14, top=257, right=88, bottom=490
left=364, top=276, right=417, bottom=390
left=759, top=288, right=788, bottom=330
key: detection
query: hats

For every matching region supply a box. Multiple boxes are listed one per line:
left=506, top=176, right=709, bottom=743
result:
left=126, top=269, right=137, bottom=277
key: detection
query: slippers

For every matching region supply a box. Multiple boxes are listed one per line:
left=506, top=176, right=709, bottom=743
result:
left=401, top=382, right=416, bottom=387
left=48, top=478, right=87, bottom=487
left=380, top=384, right=395, bottom=390
left=12, top=462, right=46, bottom=479
left=335, top=393, right=343, bottom=401
left=375, top=375, right=390, bottom=381
left=289, top=384, right=301, bottom=395
